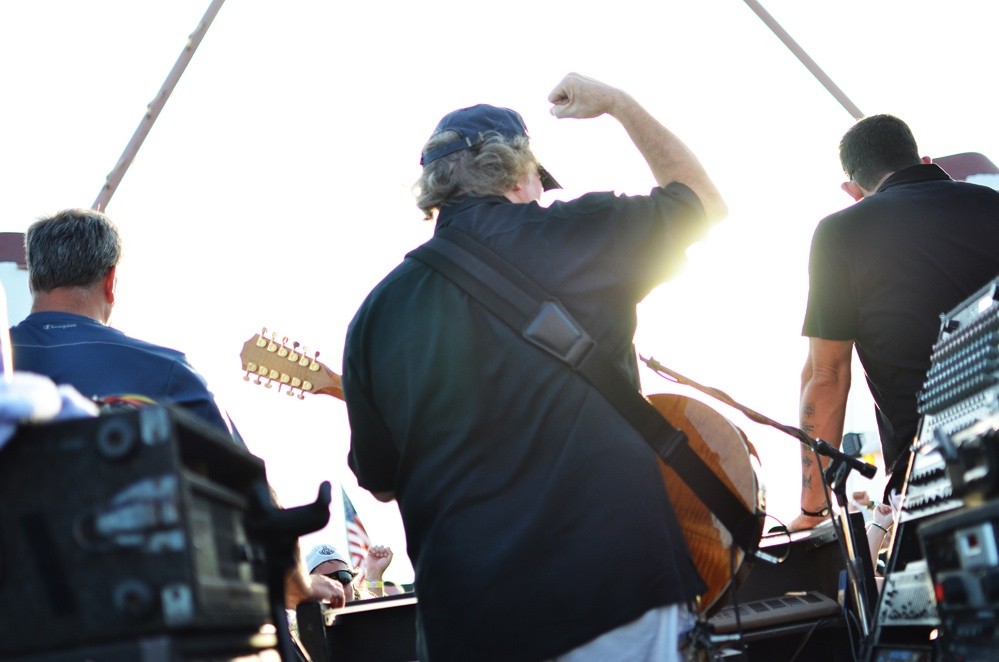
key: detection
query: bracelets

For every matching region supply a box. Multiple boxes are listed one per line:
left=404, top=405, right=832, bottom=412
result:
left=872, top=522, right=887, bottom=534
left=801, top=506, right=832, bottom=518
left=365, top=579, right=384, bottom=590
left=867, top=501, right=874, bottom=509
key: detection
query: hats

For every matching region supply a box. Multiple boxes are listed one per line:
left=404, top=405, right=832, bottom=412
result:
left=304, top=544, right=348, bottom=574
left=418, top=103, right=563, bottom=192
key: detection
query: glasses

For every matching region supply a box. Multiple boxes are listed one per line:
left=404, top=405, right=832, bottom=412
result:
left=324, top=570, right=354, bottom=585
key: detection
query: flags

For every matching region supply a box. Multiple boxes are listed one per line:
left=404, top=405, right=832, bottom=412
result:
left=343, top=488, right=372, bottom=587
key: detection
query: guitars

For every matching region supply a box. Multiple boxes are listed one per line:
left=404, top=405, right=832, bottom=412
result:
left=237, top=327, right=768, bottom=626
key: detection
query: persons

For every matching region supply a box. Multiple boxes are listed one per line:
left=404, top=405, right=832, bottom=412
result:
left=848, top=491, right=895, bottom=577
left=345, top=72, right=731, bottom=662
left=0, top=208, right=345, bottom=662
left=304, top=543, right=405, bottom=604
left=786, top=114, right=999, bottom=533
